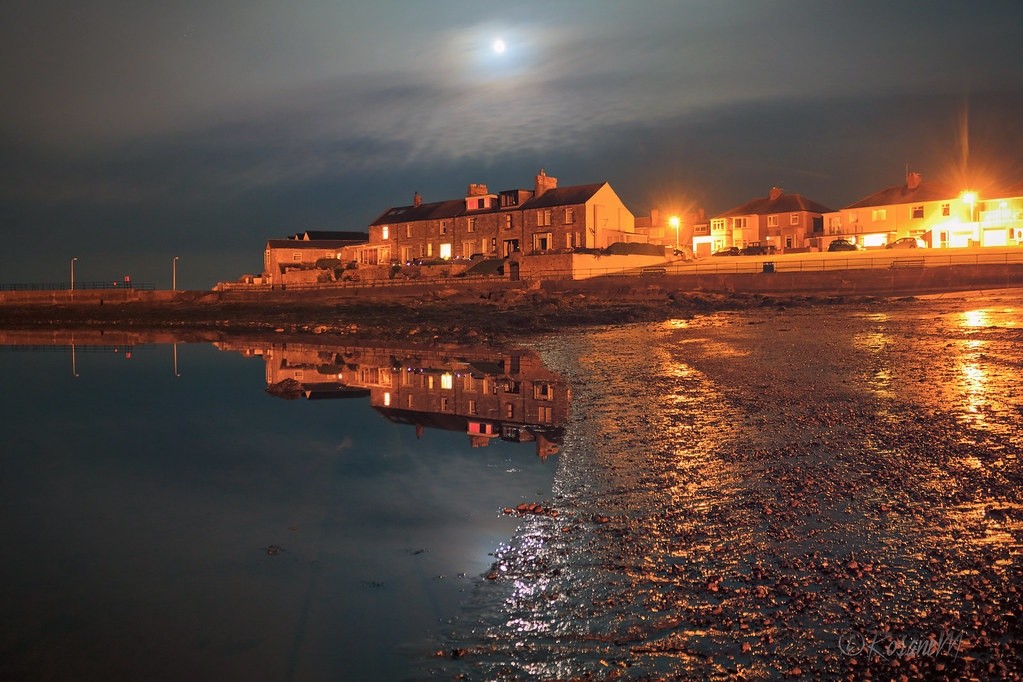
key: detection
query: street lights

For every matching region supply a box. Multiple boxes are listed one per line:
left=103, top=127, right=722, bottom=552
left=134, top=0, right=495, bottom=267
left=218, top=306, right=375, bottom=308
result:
left=960, top=190, right=978, bottom=223
left=71, top=257, right=78, bottom=290
left=669, top=216, right=681, bottom=250
left=173, top=256, right=179, bottom=291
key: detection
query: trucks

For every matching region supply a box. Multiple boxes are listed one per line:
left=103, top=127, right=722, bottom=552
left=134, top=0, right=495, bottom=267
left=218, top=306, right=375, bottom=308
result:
left=741, top=241, right=774, bottom=255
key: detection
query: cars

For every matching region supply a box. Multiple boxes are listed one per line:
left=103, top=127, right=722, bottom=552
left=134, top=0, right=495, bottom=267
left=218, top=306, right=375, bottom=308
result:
left=711, top=247, right=739, bottom=256
left=885, top=237, right=927, bottom=249
left=826, top=239, right=862, bottom=251
